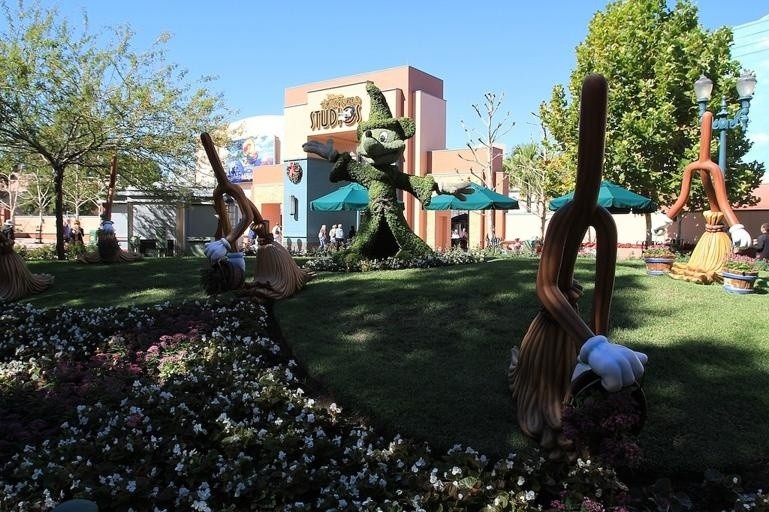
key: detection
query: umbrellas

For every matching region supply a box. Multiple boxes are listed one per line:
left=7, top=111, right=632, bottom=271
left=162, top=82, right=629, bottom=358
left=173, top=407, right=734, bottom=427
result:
left=422, top=181, right=519, bottom=210
left=549, top=179, right=658, bottom=243
left=310, top=181, right=406, bottom=231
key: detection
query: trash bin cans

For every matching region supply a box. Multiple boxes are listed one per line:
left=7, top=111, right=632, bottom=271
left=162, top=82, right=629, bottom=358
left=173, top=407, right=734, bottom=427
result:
left=140, top=240, right=157, bottom=257
left=165, top=240, right=173, bottom=256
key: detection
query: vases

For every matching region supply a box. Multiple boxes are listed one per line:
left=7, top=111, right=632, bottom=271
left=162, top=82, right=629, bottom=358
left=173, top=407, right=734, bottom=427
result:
left=643, top=254, right=676, bottom=275
left=213, top=259, right=246, bottom=289
left=721, top=267, right=758, bottom=295
left=563, top=364, right=648, bottom=436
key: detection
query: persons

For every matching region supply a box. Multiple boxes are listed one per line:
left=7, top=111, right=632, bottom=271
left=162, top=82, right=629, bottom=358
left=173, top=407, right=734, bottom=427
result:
left=318, top=224, right=328, bottom=251
left=328, top=224, right=338, bottom=249
left=460, top=227, right=469, bottom=250
left=271, top=221, right=281, bottom=243
left=752, top=223, right=769, bottom=262
left=336, top=223, right=344, bottom=251
left=510, top=237, right=522, bottom=255
left=349, top=226, right=357, bottom=238
left=451, top=228, right=459, bottom=249
left=69, top=218, right=85, bottom=245
left=63, top=217, right=73, bottom=242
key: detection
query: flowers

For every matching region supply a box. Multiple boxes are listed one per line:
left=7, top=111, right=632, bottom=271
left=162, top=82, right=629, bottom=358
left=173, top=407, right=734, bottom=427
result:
left=642, top=243, right=674, bottom=255
left=559, top=386, right=640, bottom=472
left=723, top=252, right=758, bottom=272
